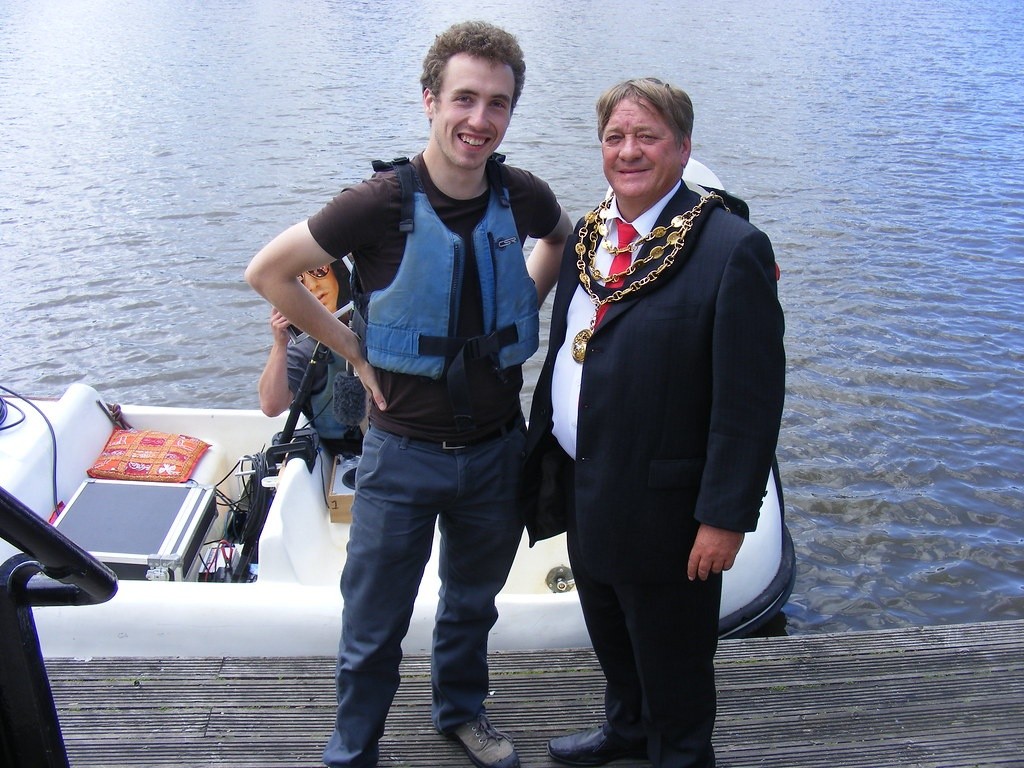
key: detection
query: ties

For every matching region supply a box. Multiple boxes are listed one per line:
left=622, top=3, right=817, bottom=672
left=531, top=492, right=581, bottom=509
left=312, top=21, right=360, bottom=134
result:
left=596, top=218, right=637, bottom=331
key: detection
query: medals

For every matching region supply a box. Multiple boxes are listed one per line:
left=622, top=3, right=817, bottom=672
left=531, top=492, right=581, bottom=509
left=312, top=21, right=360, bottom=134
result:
left=572, top=191, right=732, bottom=363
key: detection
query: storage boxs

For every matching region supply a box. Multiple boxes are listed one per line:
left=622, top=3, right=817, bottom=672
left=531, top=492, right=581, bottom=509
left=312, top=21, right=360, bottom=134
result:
left=53, top=477, right=220, bottom=585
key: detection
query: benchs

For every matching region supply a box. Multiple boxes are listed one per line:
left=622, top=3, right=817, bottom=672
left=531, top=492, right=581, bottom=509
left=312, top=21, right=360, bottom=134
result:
left=15, top=383, right=228, bottom=586
left=256, top=439, right=351, bottom=584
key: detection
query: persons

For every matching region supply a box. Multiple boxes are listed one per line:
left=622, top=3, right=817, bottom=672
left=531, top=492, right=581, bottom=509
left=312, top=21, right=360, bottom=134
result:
left=244, top=21, right=576, bottom=768
left=258, top=257, right=368, bottom=457
left=545, top=78, right=786, bottom=768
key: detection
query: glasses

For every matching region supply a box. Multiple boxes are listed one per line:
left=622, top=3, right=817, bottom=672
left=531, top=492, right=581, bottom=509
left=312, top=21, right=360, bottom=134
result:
left=298, top=263, right=330, bottom=283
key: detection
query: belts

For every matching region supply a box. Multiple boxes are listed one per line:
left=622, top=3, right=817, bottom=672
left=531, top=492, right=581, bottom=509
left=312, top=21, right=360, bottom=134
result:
left=391, top=410, right=521, bottom=445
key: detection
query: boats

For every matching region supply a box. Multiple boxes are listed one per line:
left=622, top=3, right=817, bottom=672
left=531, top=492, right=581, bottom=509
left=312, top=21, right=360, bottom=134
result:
left=0, top=160, right=801, bottom=657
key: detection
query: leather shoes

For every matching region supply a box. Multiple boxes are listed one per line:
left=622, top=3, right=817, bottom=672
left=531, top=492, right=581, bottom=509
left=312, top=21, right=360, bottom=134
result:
left=548, top=722, right=651, bottom=767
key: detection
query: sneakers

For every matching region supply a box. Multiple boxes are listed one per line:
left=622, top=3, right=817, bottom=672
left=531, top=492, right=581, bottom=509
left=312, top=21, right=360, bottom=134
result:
left=450, top=712, right=520, bottom=767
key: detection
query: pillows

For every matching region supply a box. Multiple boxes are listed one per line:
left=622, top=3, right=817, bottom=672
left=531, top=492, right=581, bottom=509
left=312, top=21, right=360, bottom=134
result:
left=89, top=428, right=214, bottom=484
